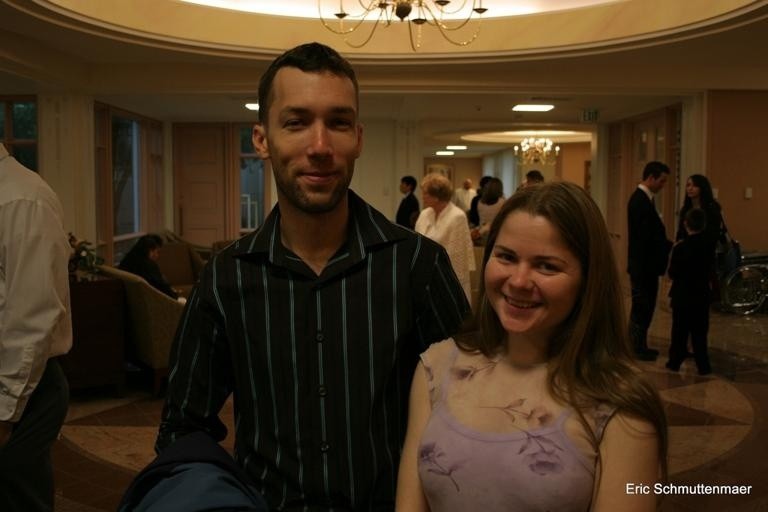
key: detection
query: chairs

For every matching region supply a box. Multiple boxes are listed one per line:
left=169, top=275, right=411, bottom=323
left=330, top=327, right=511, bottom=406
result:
left=93, top=253, right=185, bottom=401
left=156, top=230, right=235, bottom=298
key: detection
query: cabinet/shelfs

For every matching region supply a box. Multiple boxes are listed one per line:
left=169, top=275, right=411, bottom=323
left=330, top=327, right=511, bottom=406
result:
left=56, top=274, right=126, bottom=398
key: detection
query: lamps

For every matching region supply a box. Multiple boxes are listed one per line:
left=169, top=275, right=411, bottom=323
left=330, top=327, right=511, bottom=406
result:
left=315, top=0, right=488, bottom=52
left=512, top=136, right=559, bottom=167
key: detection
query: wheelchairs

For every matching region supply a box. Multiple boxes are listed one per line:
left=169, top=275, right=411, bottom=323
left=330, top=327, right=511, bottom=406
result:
left=720, top=235, right=767, bottom=315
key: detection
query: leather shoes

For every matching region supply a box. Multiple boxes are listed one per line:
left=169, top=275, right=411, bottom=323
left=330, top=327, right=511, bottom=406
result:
left=643, top=349, right=659, bottom=355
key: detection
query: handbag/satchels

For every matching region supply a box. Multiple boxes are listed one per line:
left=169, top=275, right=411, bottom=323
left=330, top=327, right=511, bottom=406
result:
left=706, top=200, right=742, bottom=281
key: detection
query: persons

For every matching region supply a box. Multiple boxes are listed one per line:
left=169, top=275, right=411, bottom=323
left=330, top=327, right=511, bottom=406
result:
left=666, top=207, right=716, bottom=375
left=394, top=172, right=423, bottom=228
left=0, top=140, right=79, bottom=510
left=675, top=175, right=723, bottom=247
left=412, top=173, right=475, bottom=318
left=154, top=42, right=479, bottom=511
left=450, top=170, right=551, bottom=250
left=121, top=229, right=190, bottom=307
left=627, top=162, right=671, bottom=359
left=395, top=179, right=664, bottom=510
